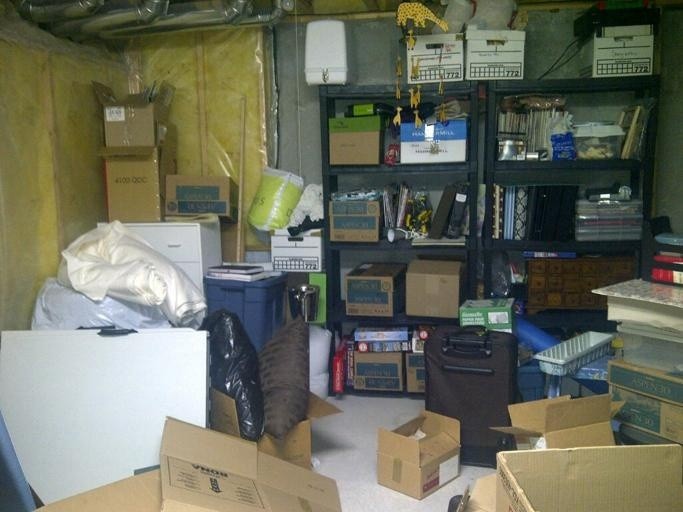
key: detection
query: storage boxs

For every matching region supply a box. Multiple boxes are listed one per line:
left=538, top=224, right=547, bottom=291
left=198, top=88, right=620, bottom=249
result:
left=399, top=121, right=467, bottom=164
left=328, top=115, right=385, bottom=168
left=464, top=354, right=682, bottom=510
left=329, top=198, right=383, bottom=243
left=92, top=122, right=180, bottom=225
left=343, top=255, right=466, bottom=395
left=404, top=29, right=464, bottom=85
left=464, top=29, right=526, bottom=82
left=38, top=416, right=345, bottom=511
left=161, top=174, right=239, bottom=206
left=165, top=202, right=237, bottom=222
left=269, top=229, right=325, bottom=274
left=374, top=407, right=462, bottom=502
left=592, top=25, right=655, bottom=79
left=210, top=387, right=343, bottom=468
left=90, top=70, right=177, bottom=148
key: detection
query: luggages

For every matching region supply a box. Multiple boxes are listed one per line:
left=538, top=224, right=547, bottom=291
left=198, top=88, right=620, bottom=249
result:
left=422, top=324, right=519, bottom=469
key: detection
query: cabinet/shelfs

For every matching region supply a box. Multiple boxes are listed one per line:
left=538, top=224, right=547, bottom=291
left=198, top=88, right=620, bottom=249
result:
left=315, top=83, right=480, bottom=403
left=487, top=70, right=662, bottom=329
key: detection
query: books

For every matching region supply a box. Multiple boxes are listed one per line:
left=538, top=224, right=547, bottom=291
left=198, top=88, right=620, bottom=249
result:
left=384, top=173, right=683, bottom=285
left=617, top=104, right=650, bottom=160
left=207, top=267, right=270, bottom=283
left=496, top=106, right=575, bottom=161
left=333, top=324, right=443, bottom=394
